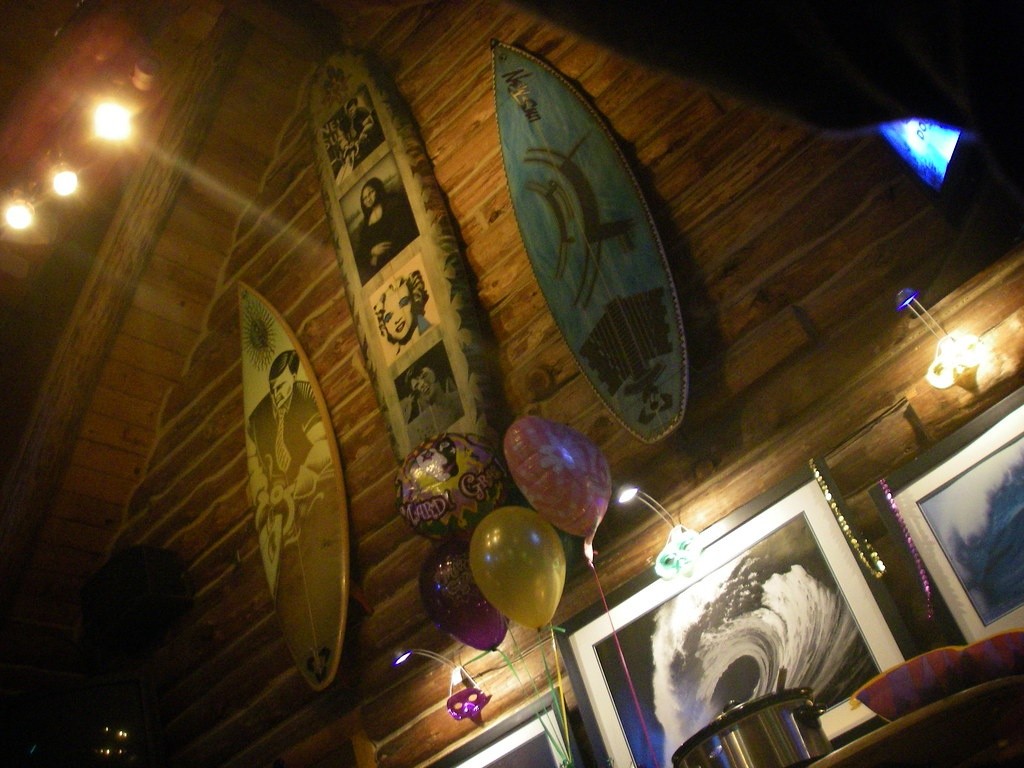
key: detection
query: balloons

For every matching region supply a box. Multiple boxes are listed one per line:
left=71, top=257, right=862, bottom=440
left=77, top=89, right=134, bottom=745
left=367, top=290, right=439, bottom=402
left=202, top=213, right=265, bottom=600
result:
left=395, top=432, right=507, bottom=539
left=470, top=506, right=566, bottom=630
left=419, top=538, right=509, bottom=651
left=503, top=417, right=609, bottom=563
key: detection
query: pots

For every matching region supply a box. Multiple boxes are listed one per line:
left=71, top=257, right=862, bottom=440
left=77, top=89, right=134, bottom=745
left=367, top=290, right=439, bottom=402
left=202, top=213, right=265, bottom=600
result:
left=672, top=687, right=834, bottom=768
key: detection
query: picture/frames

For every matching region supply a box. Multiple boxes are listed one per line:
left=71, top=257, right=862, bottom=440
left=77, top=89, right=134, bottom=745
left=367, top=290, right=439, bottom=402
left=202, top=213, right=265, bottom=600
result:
left=868, top=383, right=1024, bottom=646
left=552, top=455, right=921, bottom=768
left=425, top=687, right=587, bottom=768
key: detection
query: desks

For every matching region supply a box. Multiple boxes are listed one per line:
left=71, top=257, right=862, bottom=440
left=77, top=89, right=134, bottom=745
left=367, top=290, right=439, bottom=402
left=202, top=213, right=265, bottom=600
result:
left=808, top=675, right=1023, bottom=768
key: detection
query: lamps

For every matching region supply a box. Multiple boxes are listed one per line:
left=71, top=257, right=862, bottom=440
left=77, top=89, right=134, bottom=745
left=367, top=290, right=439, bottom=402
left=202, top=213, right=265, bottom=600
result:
left=894, top=288, right=946, bottom=335
left=392, top=648, right=457, bottom=669
